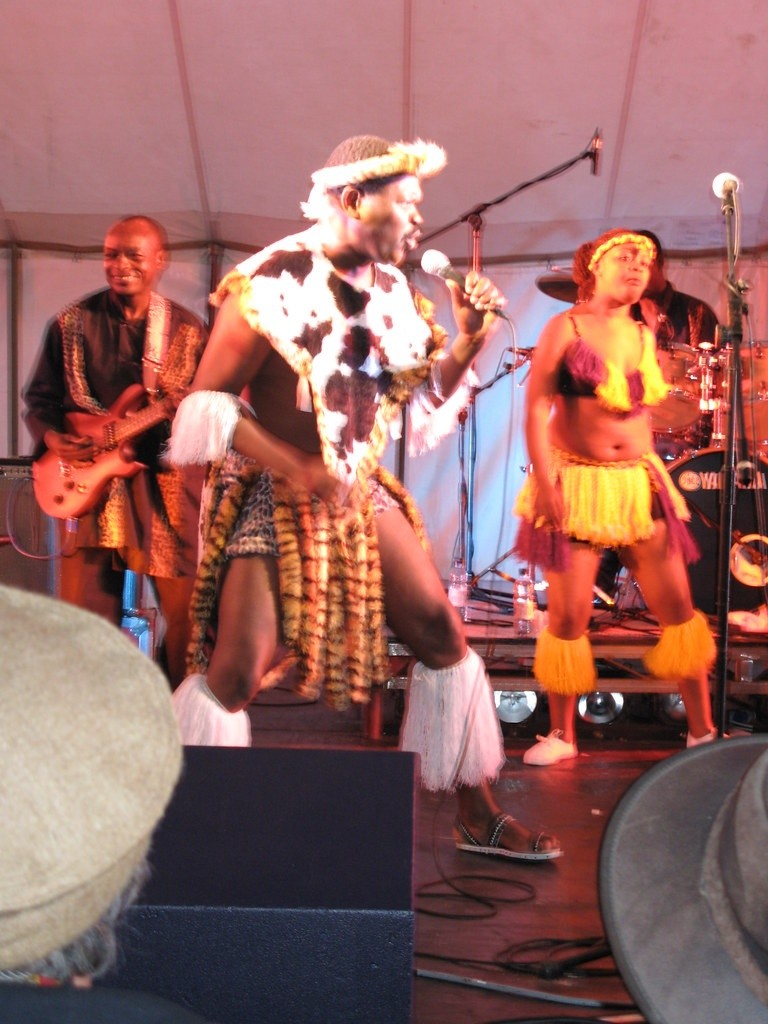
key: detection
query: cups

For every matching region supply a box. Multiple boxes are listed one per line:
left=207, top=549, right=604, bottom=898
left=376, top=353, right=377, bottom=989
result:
left=739, top=660, right=753, bottom=682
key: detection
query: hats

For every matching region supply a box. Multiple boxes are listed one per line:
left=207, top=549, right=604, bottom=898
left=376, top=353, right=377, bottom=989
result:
left=598, top=733, right=768, bottom=1024
left=0, top=585, right=183, bottom=966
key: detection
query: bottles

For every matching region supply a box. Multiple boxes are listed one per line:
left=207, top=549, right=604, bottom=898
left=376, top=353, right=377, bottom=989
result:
left=446, top=558, right=467, bottom=621
left=513, top=569, right=535, bottom=633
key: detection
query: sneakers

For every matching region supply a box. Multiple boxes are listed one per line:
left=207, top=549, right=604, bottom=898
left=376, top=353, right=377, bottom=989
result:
left=523, top=729, right=578, bottom=765
left=687, top=731, right=732, bottom=750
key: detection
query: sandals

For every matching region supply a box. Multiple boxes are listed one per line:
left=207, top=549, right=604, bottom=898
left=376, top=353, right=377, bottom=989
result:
left=455, top=810, right=561, bottom=859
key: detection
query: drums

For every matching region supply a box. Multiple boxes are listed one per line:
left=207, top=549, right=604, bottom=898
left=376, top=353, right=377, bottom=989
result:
left=650, top=342, right=768, bottom=457
left=666, top=447, right=768, bottom=617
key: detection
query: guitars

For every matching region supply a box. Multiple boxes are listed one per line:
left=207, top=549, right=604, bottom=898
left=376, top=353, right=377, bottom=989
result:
left=32, top=383, right=189, bottom=520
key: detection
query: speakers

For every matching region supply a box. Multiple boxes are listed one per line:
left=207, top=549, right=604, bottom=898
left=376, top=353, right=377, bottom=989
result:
left=94, top=742, right=418, bottom=1024
left=0, top=456, right=56, bottom=596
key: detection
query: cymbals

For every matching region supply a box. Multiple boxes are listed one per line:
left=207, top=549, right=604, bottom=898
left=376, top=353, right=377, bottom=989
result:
left=536, top=274, right=578, bottom=302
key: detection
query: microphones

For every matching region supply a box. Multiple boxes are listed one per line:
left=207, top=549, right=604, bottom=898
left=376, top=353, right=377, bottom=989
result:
left=712, top=173, right=739, bottom=198
left=421, top=248, right=509, bottom=321
left=591, top=132, right=603, bottom=176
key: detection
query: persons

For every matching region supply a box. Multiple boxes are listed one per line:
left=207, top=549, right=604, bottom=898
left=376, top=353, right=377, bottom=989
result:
left=511, top=228, right=732, bottom=765
left=171, top=134, right=564, bottom=861
left=22, top=215, right=207, bottom=679
left=593, top=230, right=720, bottom=600
